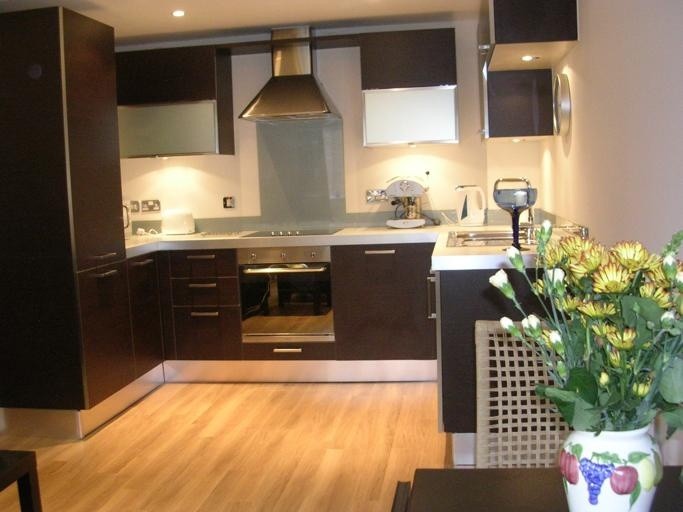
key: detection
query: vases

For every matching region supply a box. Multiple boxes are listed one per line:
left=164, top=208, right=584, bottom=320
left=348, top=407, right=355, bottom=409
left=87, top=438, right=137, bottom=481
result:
left=557, top=423, right=665, bottom=512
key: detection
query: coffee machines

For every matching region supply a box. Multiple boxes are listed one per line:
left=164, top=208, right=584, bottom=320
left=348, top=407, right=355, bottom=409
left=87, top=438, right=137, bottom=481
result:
left=386, top=179, right=426, bottom=229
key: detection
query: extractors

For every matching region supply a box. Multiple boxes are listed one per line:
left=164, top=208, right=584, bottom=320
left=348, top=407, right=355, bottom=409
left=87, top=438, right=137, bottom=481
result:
left=236, top=25, right=342, bottom=123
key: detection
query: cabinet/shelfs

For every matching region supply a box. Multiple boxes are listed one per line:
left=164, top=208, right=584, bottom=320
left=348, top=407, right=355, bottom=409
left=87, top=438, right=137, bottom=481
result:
left=1, top=7, right=127, bottom=271
left=168, top=247, right=436, bottom=361
left=477, top=0, right=580, bottom=73
left=4, top=253, right=166, bottom=415
left=355, top=28, right=458, bottom=147
left=427, top=269, right=575, bottom=433
left=115, top=47, right=237, bottom=160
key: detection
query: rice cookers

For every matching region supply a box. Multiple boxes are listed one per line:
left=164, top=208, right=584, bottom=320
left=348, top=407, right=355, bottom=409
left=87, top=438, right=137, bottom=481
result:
left=161, top=208, right=195, bottom=234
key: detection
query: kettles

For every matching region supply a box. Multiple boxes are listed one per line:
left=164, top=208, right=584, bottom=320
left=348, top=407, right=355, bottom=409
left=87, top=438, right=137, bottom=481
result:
left=455, top=184, right=486, bottom=226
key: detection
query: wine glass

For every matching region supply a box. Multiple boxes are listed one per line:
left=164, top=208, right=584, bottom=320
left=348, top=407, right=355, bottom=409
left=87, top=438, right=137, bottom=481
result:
left=494, top=188, right=538, bottom=251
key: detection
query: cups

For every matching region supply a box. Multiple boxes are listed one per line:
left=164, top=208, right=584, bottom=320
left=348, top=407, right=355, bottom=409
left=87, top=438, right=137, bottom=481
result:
left=405, top=205, right=416, bottom=218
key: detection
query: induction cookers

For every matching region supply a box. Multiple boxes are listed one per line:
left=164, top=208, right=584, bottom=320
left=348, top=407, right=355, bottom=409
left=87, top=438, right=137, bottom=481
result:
left=243, top=228, right=342, bottom=237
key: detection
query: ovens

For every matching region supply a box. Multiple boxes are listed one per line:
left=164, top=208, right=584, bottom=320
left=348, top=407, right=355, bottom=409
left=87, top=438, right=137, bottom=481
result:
left=235, top=249, right=335, bottom=344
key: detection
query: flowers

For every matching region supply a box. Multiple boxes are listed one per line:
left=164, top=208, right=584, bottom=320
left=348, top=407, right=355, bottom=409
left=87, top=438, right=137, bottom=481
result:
left=486, top=216, right=683, bottom=431
